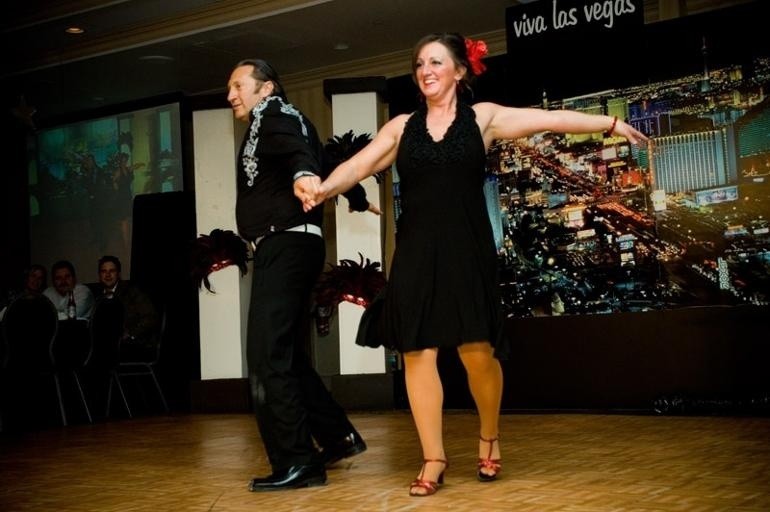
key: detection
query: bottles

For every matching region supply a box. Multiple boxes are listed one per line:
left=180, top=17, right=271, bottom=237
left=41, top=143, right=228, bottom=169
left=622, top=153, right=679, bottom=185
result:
left=67, top=288, right=76, bottom=320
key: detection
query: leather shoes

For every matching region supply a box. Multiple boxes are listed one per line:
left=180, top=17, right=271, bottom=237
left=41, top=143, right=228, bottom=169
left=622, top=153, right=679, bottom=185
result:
left=249, top=459, right=326, bottom=491
left=320, top=430, right=367, bottom=468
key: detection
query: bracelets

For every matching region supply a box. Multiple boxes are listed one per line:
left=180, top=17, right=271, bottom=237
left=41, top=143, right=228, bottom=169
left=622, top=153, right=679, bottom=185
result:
left=608, top=114, right=620, bottom=139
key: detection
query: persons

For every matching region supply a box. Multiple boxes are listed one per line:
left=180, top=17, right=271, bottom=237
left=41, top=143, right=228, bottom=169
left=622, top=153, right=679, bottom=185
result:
left=302, top=31, right=655, bottom=499
left=69, top=153, right=120, bottom=248
left=110, top=150, right=146, bottom=251
left=89, top=254, right=155, bottom=367
left=9, top=266, right=50, bottom=302
left=42, top=259, right=98, bottom=365
left=225, top=57, right=386, bottom=493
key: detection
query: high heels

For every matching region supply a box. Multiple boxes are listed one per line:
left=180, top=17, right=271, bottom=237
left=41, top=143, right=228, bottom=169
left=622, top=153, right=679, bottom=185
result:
left=409, top=456, right=449, bottom=496
left=477, top=436, right=501, bottom=481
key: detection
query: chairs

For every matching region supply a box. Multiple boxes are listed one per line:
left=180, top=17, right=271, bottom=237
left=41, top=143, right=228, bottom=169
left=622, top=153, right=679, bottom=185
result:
left=0, top=291, right=171, bottom=426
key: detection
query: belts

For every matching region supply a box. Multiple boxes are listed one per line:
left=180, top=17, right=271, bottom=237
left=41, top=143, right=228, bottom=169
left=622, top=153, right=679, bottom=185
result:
left=249, top=224, right=322, bottom=252
